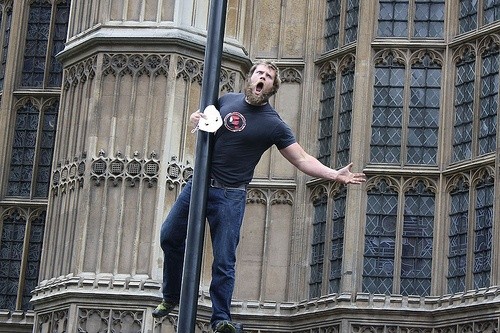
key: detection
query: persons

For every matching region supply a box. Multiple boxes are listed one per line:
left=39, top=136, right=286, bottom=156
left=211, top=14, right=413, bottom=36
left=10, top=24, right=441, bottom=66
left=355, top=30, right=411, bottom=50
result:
left=152, top=58, right=368, bottom=333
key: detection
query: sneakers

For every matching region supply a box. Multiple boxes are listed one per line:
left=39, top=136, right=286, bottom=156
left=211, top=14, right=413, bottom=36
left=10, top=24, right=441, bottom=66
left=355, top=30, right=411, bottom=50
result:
left=212, top=320, right=236, bottom=333
left=151, top=298, right=180, bottom=319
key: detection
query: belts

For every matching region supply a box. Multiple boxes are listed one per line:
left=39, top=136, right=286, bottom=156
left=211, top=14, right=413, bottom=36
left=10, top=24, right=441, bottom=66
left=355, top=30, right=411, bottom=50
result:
left=210, top=179, right=246, bottom=193
